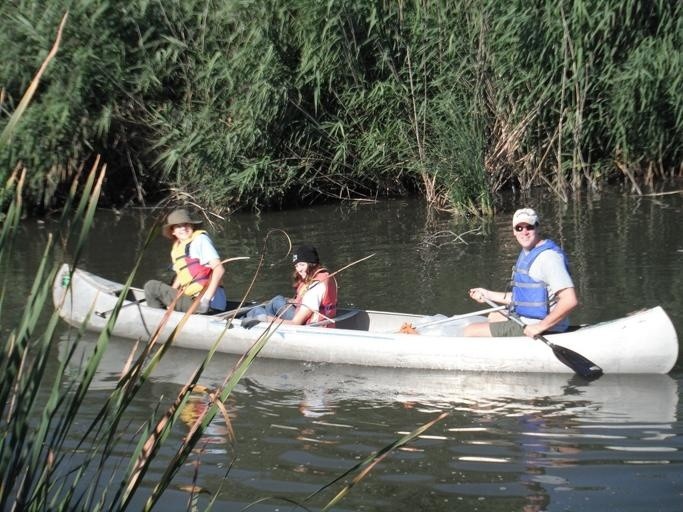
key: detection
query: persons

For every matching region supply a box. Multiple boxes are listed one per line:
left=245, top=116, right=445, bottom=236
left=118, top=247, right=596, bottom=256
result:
left=468, top=399, right=583, bottom=511
left=254, top=386, right=340, bottom=452
left=141, top=208, right=226, bottom=316
left=144, top=379, right=235, bottom=512
left=240, top=244, right=339, bottom=330
left=455, top=206, right=578, bottom=340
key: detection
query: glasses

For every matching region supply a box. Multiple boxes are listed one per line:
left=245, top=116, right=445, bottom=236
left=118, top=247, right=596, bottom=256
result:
left=513, top=224, right=534, bottom=232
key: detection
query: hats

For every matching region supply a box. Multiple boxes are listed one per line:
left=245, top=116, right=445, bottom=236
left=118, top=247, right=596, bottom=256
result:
left=512, top=207, right=542, bottom=228
left=161, top=209, right=203, bottom=240
left=292, top=245, right=320, bottom=266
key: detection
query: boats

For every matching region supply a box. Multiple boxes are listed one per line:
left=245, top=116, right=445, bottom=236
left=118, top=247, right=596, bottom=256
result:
left=52, top=262, right=679, bottom=374
left=58, top=328, right=679, bottom=464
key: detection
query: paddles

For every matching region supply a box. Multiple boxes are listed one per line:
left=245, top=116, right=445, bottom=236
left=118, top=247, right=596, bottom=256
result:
left=484, top=296, right=603, bottom=380
